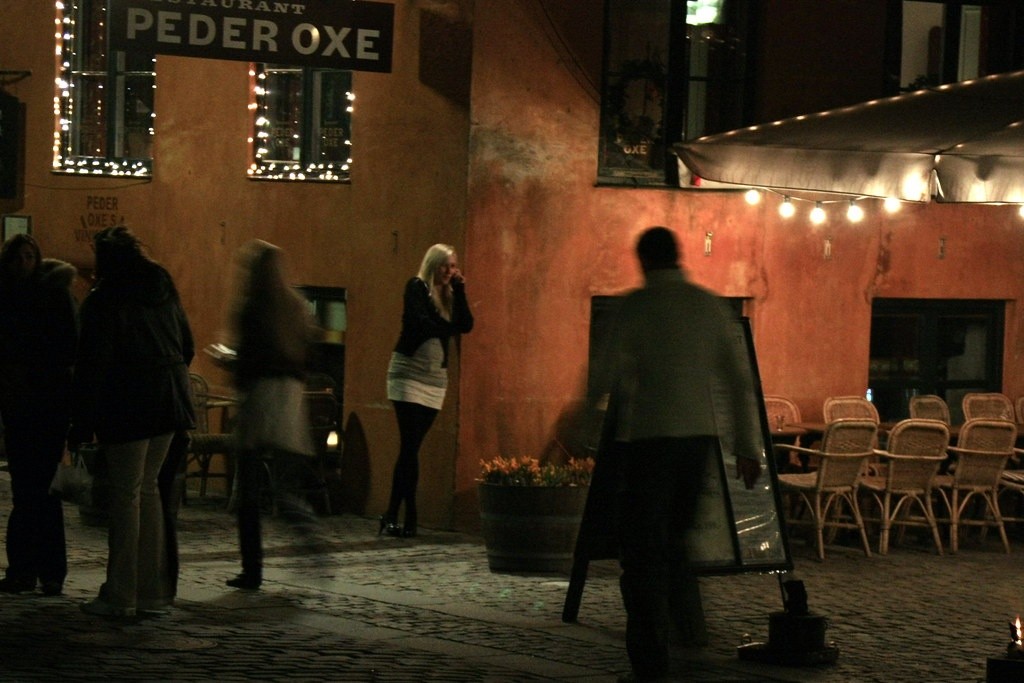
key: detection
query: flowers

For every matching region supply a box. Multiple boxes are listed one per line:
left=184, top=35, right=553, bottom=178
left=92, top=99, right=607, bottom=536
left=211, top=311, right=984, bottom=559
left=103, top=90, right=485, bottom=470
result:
left=478, top=455, right=595, bottom=489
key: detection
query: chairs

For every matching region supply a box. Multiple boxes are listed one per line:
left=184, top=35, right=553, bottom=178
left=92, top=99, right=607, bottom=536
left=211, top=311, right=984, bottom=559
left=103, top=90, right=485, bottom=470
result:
left=185, top=374, right=240, bottom=498
left=763, top=392, right=1022, bottom=561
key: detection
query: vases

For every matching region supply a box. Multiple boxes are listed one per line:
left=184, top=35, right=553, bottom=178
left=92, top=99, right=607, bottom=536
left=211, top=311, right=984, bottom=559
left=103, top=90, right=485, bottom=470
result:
left=476, top=483, right=591, bottom=574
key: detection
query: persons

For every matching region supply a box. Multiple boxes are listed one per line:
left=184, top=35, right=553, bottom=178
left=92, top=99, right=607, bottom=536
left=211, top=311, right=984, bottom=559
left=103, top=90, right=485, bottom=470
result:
left=213, top=239, right=317, bottom=592
left=0, top=234, right=78, bottom=597
left=377, top=243, right=473, bottom=537
left=75, top=225, right=195, bottom=619
left=575, top=227, right=761, bottom=683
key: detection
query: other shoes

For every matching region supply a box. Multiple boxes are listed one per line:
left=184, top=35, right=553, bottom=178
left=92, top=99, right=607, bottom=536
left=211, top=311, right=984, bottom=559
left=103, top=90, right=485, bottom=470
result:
left=78, top=597, right=137, bottom=618
left=40, top=579, right=62, bottom=595
left=0, top=568, right=33, bottom=595
left=614, top=671, right=640, bottom=683
left=225, top=574, right=262, bottom=591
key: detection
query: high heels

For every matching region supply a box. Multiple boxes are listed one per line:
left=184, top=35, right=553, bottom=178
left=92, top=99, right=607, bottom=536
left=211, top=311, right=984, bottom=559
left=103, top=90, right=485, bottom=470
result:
left=402, top=522, right=417, bottom=538
left=378, top=513, right=401, bottom=537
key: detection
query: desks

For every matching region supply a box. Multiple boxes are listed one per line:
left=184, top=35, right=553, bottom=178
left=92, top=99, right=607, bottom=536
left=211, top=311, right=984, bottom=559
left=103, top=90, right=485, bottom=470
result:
left=764, top=428, right=807, bottom=540
left=775, top=419, right=1021, bottom=546
